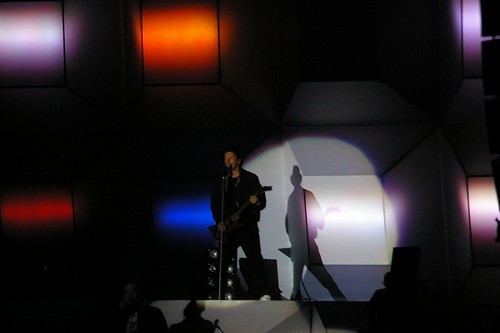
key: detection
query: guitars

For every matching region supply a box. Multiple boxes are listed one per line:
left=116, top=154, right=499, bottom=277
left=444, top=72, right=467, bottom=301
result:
left=207, top=186, right=273, bottom=241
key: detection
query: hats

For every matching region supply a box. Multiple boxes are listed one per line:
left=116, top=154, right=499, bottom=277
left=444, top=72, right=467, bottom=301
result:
left=221, top=134, right=239, bottom=154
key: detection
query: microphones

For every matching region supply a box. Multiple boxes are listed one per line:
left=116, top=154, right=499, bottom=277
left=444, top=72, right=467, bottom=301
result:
left=227, top=164, right=232, bottom=176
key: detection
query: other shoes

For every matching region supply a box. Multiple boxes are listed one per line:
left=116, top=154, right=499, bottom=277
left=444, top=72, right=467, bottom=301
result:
left=259, top=294, right=272, bottom=301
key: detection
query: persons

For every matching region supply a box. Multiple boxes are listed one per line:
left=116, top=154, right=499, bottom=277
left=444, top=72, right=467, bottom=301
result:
left=369, top=272, right=397, bottom=333
left=209, top=147, right=272, bottom=301
left=109, top=282, right=219, bottom=333
